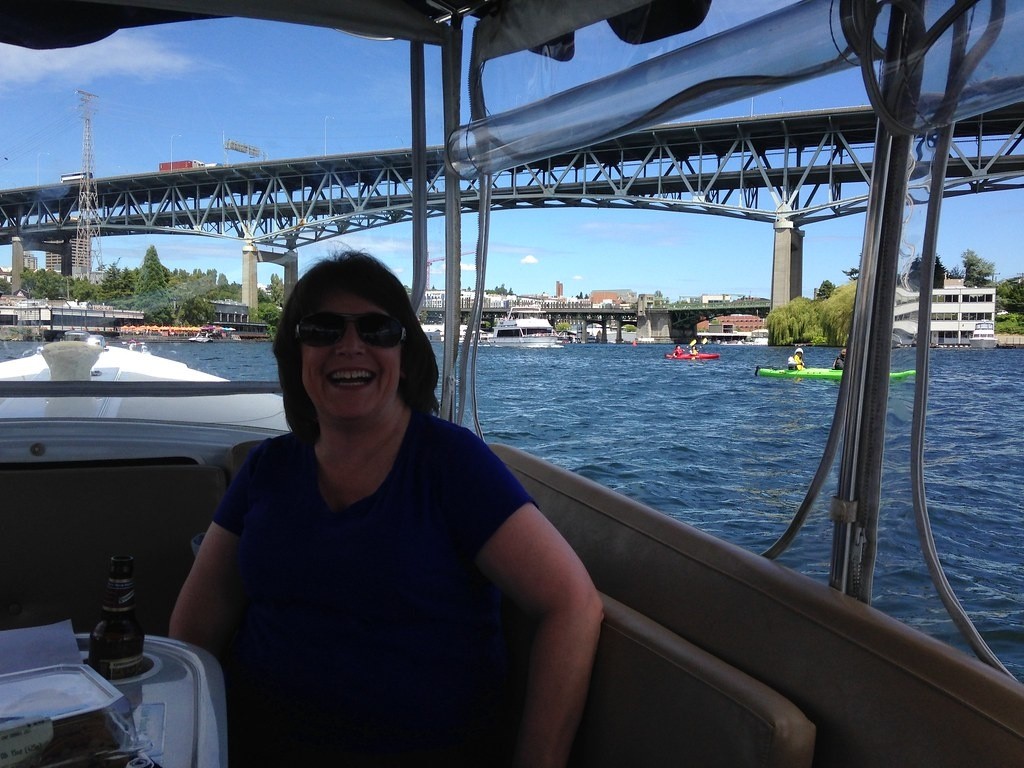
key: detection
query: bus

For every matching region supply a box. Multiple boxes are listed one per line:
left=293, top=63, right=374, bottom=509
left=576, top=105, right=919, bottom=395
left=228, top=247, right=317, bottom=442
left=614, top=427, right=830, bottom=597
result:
left=60, top=172, right=93, bottom=183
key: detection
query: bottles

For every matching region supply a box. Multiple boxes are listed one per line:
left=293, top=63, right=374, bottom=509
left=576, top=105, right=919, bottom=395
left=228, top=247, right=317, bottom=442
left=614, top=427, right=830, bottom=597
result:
left=89, top=555, right=145, bottom=680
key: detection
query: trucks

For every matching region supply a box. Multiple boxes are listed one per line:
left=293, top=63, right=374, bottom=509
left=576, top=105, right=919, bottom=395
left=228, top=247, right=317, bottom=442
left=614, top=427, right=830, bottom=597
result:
left=159, top=160, right=205, bottom=172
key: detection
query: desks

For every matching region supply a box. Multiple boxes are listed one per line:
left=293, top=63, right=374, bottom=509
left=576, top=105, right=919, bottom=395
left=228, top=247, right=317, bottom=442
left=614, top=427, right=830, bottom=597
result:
left=0, top=633, right=228, bottom=768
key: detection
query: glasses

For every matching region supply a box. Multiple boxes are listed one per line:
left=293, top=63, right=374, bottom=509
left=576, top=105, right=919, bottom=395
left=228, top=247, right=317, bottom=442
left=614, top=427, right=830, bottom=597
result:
left=293, top=312, right=406, bottom=348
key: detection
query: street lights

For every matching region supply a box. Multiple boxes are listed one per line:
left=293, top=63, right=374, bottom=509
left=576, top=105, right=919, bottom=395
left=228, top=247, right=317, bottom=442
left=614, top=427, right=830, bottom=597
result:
left=169, top=133, right=182, bottom=172
left=37, top=152, right=51, bottom=187
left=324, top=115, right=335, bottom=158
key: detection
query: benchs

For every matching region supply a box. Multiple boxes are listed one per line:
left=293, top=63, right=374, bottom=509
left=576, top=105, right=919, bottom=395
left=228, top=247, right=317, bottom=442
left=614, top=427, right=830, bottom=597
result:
left=562, top=590, right=816, bottom=768
left=0, top=466, right=227, bottom=637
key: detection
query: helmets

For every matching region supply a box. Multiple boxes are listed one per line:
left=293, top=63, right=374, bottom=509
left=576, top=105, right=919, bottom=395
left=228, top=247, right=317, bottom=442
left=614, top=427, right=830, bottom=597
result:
left=794, top=348, right=804, bottom=355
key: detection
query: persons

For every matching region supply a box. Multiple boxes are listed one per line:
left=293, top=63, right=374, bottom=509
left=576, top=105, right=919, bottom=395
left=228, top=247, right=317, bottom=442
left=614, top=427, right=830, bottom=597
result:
left=687, top=345, right=703, bottom=355
left=794, top=348, right=806, bottom=370
left=168, top=249, right=606, bottom=768
left=832, top=348, right=846, bottom=370
left=204, top=331, right=210, bottom=338
left=673, top=344, right=685, bottom=359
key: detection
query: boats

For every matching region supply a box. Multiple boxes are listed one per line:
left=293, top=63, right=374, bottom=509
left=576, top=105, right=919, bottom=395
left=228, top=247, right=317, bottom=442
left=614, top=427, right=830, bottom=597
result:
left=122, top=339, right=146, bottom=347
left=665, top=352, right=720, bottom=360
left=486, top=306, right=564, bottom=349
left=753, top=365, right=916, bottom=384
left=188, top=324, right=242, bottom=344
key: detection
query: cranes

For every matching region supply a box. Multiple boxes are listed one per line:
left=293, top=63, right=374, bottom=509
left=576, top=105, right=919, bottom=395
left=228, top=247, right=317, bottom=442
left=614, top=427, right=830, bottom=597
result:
left=425, top=250, right=476, bottom=290
